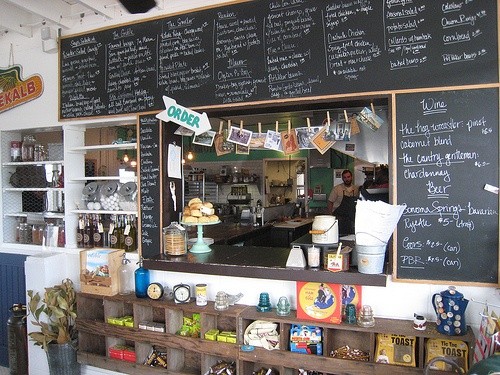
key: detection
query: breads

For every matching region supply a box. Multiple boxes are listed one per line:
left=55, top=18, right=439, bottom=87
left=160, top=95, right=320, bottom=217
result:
left=183, top=198, right=219, bottom=223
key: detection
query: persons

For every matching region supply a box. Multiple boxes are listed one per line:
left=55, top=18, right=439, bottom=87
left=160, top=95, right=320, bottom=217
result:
left=328, top=170, right=360, bottom=236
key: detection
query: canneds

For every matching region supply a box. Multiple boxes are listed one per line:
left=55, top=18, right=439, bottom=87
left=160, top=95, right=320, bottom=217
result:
left=195, top=284, right=207, bottom=306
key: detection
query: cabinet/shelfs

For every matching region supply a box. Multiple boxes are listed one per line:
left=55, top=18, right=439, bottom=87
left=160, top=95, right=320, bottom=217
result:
left=184, top=173, right=219, bottom=203
left=75, top=291, right=476, bottom=375
left=0, top=115, right=143, bottom=260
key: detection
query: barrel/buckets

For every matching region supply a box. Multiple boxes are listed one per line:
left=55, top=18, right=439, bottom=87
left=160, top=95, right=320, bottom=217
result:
left=309, top=215, right=338, bottom=243
left=354, top=232, right=387, bottom=274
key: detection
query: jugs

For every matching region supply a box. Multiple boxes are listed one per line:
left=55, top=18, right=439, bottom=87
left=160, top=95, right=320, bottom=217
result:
left=432, top=286, right=469, bottom=336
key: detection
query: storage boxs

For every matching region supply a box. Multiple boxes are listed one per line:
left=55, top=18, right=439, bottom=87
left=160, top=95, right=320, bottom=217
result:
left=78, top=248, right=125, bottom=297
left=107, top=316, right=134, bottom=328
left=139, top=321, right=165, bottom=332
left=109, top=344, right=136, bottom=362
left=205, top=329, right=236, bottom=343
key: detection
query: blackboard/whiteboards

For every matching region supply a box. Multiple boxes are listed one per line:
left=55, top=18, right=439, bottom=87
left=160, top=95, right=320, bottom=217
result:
left=57, top=0, right=500, bottom=122
left=390, top=83, right=499, bottom=290
left=136, top=111, right=163, bottom=258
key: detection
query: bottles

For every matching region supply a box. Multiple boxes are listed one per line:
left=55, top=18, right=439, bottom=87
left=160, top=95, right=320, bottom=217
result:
left=34, top=142, right=46, bottom=161
left=10, top=140, right=22, bottom=162
left=15, top=217, right=65, bottom=247
left=117, top=253, right=151, bottom=298
left=256, top=202, right=262, bottom=226
left=76, top=213, right=138, bottom=252
left=22, top=134, right=38, bottom=161
left=189, top=167, right=207, bottom=181
left=226, top=174, right=257, bottom=183
left=81, top=181, right=138, bottom=211
left=52, top=170, right=61, bottom=188
left=163, top=221, right=187, bottom=256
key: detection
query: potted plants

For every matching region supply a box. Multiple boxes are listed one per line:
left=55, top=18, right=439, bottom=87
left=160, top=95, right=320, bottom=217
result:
left=21, top=278, right=81, bottom=375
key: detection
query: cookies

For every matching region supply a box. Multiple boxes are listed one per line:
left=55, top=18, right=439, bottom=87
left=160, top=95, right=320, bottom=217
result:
left=165, top=234, right=186, bottom=255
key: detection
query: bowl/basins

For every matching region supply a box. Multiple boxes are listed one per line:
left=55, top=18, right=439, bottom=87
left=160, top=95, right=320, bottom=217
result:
left=339, top=238, right=357, bottom=267
left=272, top=180, right=280, bottom=186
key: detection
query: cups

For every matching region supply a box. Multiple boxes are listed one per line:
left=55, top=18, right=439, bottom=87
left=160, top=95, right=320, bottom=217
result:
left=413, top=314, right=427, bottom=331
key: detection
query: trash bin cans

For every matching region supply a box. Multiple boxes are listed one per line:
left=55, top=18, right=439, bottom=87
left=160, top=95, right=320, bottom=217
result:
left=7, top=305, right=28, bottom=375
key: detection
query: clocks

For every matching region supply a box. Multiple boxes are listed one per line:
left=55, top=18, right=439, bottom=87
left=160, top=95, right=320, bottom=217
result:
left=146, top=283, right=164, bottom=301
left=173, top=284, right=190, bottom=304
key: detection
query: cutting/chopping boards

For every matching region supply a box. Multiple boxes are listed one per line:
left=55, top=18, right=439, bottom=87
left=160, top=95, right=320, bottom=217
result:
left=273, top=218, right=313, bottom=228
